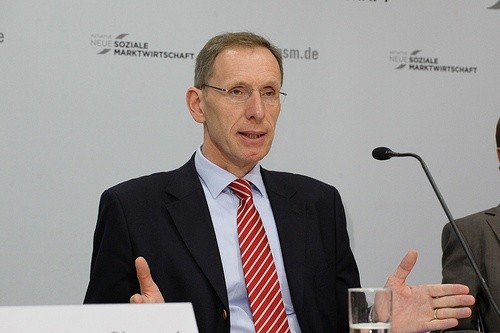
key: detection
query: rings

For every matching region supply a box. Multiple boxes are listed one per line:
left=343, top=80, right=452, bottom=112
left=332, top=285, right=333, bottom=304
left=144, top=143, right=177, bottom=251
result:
left=433, top=309, right=439, bottom=319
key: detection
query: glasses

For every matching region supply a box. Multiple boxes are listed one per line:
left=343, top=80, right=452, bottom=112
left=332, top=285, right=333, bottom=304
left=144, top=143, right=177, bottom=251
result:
left=202, top=83, right=288, bottom=106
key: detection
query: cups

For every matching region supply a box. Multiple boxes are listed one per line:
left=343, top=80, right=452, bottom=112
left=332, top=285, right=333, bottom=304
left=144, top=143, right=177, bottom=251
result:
left=348, top=288, right=393, bottom=333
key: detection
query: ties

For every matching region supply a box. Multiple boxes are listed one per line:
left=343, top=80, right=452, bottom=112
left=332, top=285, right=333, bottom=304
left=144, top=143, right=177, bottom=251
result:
left=227, top=179, right=291, bottom=333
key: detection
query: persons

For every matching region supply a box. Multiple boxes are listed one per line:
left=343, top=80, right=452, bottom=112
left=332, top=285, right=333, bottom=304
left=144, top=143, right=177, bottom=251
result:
left=83, top=32, right=475, bottom=333
left=441, top=119, right=500, bottom=333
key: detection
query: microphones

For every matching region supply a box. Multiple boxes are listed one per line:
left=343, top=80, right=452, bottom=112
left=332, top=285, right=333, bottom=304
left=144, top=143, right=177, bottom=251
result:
left=373, top=147, right=500, bottom=315
